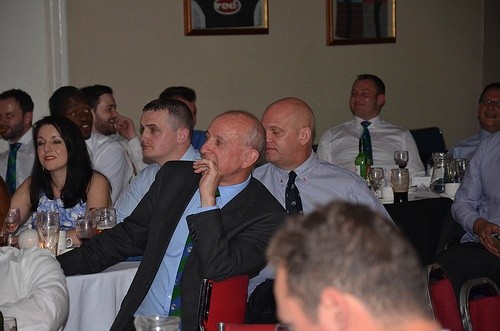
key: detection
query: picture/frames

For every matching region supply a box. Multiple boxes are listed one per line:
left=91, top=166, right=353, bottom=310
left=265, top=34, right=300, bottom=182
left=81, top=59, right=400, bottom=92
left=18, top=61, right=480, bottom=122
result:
left=183, top=0, right=269, bottom=37
left=325, top=0, right=396, bottom=45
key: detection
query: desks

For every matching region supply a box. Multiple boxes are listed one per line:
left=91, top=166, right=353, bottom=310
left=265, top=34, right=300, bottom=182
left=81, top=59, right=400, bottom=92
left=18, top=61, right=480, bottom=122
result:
left=373, top=179, right=455, bottom=202
left=65, top=259, right=140, bottom=331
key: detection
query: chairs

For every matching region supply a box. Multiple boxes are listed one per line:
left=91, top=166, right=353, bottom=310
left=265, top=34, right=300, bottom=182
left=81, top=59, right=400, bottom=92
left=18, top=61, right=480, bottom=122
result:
left=198, top=274, right=249, bottom=331
left=382, top=198, right=452, bottom=260
left=424, top=263, right=462, bottom=331
left=407, top=127, right=445, bottom=176
left=460, top=277, right=500, bottom=331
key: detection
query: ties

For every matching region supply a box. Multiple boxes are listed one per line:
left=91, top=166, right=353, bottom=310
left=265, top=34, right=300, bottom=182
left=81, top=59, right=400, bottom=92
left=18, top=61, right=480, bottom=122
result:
left=358, top=121, right=373, bottom=166
left=4, top=142, right=22, bottom=197
left=284, top=171, right=304, bottom=217
left=168, top=188, right=219, bottom=331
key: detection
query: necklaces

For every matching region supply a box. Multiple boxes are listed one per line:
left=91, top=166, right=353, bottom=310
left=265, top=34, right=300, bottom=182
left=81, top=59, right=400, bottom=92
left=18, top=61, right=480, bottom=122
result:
left=50, top=175, right=64, bottom=191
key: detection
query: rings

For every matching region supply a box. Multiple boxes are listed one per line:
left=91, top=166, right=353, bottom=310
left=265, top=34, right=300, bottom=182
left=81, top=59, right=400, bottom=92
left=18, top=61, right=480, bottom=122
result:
left=483, top=236, right=486, bottom=241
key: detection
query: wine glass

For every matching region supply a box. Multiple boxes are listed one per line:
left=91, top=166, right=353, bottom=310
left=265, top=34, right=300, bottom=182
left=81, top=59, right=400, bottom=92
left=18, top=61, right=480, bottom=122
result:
left=368, top=168, right=384, bottom=200
left=394, top=151, right=409, bottom=184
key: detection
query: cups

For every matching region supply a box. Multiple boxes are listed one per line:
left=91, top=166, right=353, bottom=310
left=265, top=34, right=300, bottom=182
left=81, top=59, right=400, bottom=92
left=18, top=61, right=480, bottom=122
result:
left=3, top=233, right=19, bottom=249
left=1, top=208, right=20, bottom=232
left=0, top=317, right=17, bottom=331
left=134, top=315, right=182, bottom=331
left=409, top=178, right=412, bottom=188
left=58, top=230, right=73, bottom=250
left=381, top=187, right=394, bottom=202
left=36, top=213, right=60, bottom=256
left=430, top=152, right=469, bottom=197
left=75, top=206, right=117, bottom=242
left=391, top=169, right=409, bottom=203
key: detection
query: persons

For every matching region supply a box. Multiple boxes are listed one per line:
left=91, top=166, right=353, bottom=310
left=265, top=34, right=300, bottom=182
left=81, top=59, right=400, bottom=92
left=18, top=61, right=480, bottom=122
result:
left=450, top=131, right=500, bottom=300
left=317, top=73, right=426, bottom=189
left=448, top=81, right=500, bottom=162
left=266, top=203, right=451, bottom=331
left=0, top=84, right=397, bottom=331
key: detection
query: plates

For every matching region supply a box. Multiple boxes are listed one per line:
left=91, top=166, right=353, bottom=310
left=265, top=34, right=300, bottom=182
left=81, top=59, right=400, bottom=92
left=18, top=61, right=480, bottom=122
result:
left=408, top=187, right=417, bottom=192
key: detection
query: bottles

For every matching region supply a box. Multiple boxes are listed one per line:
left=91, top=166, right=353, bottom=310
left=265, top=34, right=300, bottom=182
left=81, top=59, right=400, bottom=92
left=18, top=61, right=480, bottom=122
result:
left=354, top=138, right=371, bottom=191
